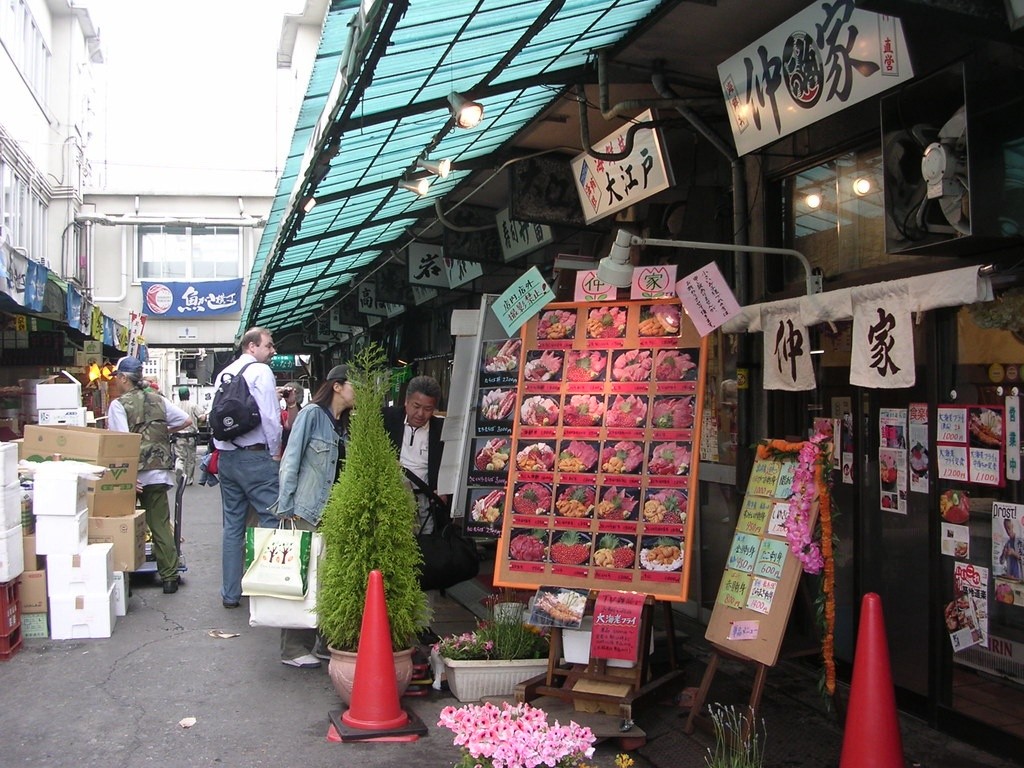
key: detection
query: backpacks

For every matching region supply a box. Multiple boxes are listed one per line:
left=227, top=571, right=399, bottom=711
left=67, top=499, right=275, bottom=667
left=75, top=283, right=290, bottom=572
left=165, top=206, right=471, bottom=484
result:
left=208, top=361, right=262, bottom=442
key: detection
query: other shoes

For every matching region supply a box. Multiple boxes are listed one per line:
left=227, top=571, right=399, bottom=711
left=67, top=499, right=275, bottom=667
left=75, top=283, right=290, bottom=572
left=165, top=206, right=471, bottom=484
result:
left=317, top=654, right=330, bottom=660
left=282, top=653, right=322, bottom=668
left=163, top=576, right=181, bottom=593
left=176, top=468, right=183, bottom=484
left=187, top=481, right=192, bottom=485
left=223, top=602, right=239, bottom=607
left=417, top=629, right=441, bottom=645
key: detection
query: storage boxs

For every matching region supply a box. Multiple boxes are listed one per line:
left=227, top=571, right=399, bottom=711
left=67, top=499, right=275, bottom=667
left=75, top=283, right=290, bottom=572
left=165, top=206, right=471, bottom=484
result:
left=0, top=341, right=146, bottom=661
left=562, top=615, right=655, bottom=668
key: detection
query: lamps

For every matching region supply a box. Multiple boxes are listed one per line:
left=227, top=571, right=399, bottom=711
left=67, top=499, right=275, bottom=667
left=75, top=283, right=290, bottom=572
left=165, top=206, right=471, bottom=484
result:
left=415, top=157, right=450, bottom=178
left=805, top=193, right=823, bottom=210
left=93, top=361, right=101, bottom=377
left=446, top=91, right=483, bottom=129
left=398, top=179, right=429, bottom=196
left=90, top=363, right=97, bottom=381
left=597, top=228, right=823, bottom=293
left=854, top=176, right=877, bottom=197
left=299, top=195, right=317, bottom=214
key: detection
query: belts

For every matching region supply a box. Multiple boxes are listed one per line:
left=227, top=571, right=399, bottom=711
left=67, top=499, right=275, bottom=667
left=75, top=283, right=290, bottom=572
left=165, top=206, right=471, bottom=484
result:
left=244, top=444, right=268, bottom=450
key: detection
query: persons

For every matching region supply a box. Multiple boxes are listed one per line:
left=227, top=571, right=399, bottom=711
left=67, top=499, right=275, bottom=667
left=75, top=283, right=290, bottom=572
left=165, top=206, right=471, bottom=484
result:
left=276, top=380, right=304, bottom=462
left=382, top=376, right=453, bottom=553
left=170, top=387, right=206, bottom=487
left=999, top=519, right=1024, bottom=579
left=208, top=326, right=284, bottom=608
left=106, top=356, right=192, bottom=594
left=267, top=364, right=362, bottom=669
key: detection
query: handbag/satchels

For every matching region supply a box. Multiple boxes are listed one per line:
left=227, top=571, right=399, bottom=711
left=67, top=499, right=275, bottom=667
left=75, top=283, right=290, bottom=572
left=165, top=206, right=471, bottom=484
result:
left=417, top=505, right=481, bottom=592
left=241, top=517, right=328, bottom=629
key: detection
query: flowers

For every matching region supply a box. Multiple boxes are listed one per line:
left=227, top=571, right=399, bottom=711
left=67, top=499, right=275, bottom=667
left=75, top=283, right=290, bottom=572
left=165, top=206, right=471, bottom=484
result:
left=704, top=703, right=768, bottom=768
left=778, top=430, right=836, bottom=574
left=429, top=587, right=551, bottom=660
left=437, top=701, right=636, bottom=768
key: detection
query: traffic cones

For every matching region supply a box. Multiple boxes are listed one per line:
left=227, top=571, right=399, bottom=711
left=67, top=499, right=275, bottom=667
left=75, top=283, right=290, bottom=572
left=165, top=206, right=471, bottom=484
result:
left=324, top=570, right=430, bottom=745
left=838, top=591, right=905, bottom=768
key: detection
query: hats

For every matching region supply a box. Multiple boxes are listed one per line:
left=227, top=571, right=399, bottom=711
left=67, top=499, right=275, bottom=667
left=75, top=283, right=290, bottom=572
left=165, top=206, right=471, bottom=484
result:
left=108, top=355, right=142, bottom=376
left=326, top=364, right=349, bottom=379
left=179, top=388, right=189, bottom=396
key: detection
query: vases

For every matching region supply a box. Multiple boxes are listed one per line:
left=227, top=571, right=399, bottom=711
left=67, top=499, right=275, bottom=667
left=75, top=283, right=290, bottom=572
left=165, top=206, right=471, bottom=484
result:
left=440, top=655, right=569, bottom=702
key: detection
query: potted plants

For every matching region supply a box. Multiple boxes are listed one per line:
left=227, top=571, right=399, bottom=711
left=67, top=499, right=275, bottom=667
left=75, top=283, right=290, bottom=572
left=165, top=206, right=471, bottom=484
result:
left=304, top=340, right=436, bottom=709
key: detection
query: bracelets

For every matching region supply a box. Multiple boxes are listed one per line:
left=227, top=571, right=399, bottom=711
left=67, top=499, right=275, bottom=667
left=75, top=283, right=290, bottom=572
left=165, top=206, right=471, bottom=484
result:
left=289, top=402, right=296, bottom=409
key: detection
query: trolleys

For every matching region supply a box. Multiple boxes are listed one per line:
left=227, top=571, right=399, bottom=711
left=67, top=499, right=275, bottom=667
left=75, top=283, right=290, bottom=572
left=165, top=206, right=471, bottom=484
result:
left=131, top=473, right=187, bottom=582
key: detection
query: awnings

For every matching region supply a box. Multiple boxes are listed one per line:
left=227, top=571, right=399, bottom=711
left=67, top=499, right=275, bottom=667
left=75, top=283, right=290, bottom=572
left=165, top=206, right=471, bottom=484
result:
left=236, top=0, right=674, bottom=344
